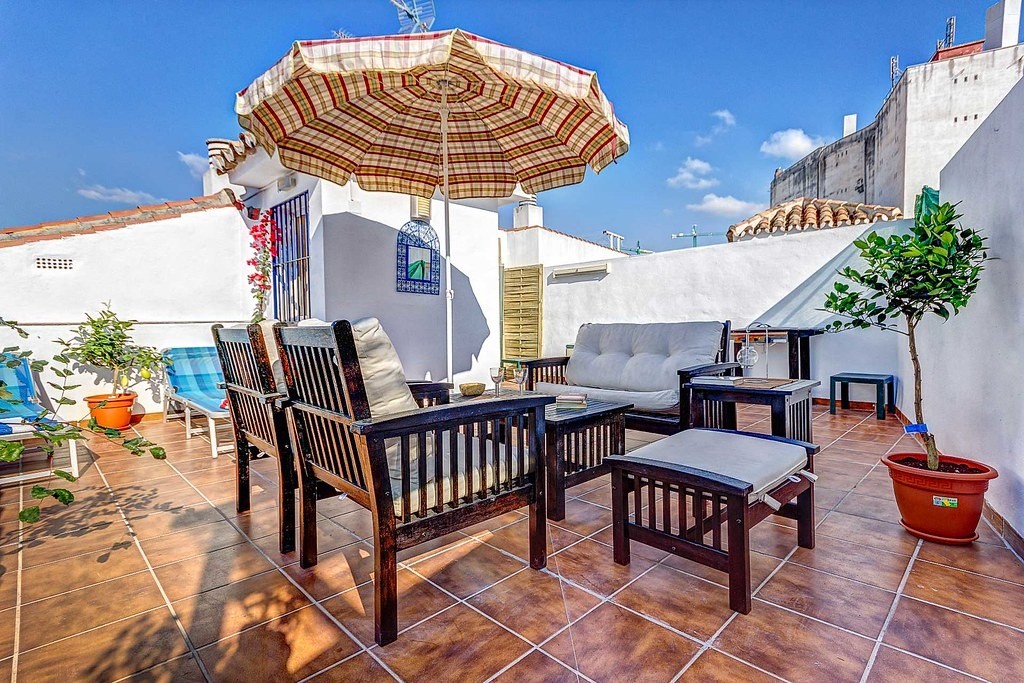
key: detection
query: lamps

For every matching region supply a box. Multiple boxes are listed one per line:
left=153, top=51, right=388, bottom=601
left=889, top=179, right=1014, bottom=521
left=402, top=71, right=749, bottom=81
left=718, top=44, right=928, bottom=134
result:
left=409, top=195, right=431, bottom=224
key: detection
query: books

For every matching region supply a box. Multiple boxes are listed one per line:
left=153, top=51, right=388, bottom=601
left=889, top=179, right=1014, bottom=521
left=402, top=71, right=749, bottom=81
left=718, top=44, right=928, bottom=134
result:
left=556, top=393, right=588, bottom=409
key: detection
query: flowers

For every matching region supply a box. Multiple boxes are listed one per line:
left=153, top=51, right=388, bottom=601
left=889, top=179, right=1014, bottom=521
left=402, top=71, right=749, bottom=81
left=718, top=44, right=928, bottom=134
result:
left=245, top=207, right=283, bottom=292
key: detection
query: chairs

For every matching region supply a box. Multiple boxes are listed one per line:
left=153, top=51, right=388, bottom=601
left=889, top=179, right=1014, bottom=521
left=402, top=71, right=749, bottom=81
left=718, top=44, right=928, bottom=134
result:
left=271, top=321, right=558, bottom=648
left=161, top=345, right=254, bottom=459
left=0, top=353, right=79, bottom=483
left=209, top=322, right=321, bottom=554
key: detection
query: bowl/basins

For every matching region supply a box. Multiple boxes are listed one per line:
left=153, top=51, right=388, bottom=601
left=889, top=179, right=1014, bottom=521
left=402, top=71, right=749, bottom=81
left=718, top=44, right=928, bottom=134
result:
left=460, top=383, right=485, bottom=396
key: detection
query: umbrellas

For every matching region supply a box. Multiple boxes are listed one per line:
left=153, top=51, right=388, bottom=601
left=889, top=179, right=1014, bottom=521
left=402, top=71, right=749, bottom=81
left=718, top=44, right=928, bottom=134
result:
left=234, top=28, right=629, bottom=395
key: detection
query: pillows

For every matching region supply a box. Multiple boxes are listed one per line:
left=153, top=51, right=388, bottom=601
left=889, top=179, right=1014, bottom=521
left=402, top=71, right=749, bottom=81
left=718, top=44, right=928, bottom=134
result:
left=230, top=317, right=286, bottom=392
left=298, top=316, right=434, bottom=484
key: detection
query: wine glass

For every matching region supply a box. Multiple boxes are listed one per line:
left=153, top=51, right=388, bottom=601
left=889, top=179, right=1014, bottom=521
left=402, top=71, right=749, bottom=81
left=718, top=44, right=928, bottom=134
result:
left=490, top=368, right=505, bottom=398
left=513, top=368, right=529, bottom=398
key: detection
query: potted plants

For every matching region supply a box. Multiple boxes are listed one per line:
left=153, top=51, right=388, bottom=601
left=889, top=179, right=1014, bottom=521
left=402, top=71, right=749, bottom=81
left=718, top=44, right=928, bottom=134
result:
left=812, top=198, right=1003, bottom=545
left=50, top=298, right=173, bottom=431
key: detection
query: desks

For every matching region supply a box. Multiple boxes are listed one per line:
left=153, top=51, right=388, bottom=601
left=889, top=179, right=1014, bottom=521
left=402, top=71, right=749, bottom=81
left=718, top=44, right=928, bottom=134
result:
left=448, top=389, right=634, bottom=521
left=729, top=327, right=824, bottom=381
left=682, top=375, right=820, bottom=444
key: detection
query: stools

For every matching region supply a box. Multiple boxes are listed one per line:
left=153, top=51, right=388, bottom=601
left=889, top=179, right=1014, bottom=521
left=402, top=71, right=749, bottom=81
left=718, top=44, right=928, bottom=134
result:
left=828, top=371, right=896, bottom=420
left=602, top=426, right=821, bottom=615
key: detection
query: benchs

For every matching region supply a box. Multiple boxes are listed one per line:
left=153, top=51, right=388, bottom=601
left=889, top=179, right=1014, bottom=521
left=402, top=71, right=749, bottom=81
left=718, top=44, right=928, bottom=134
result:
left=520, top=320, right=732, bottom=436
